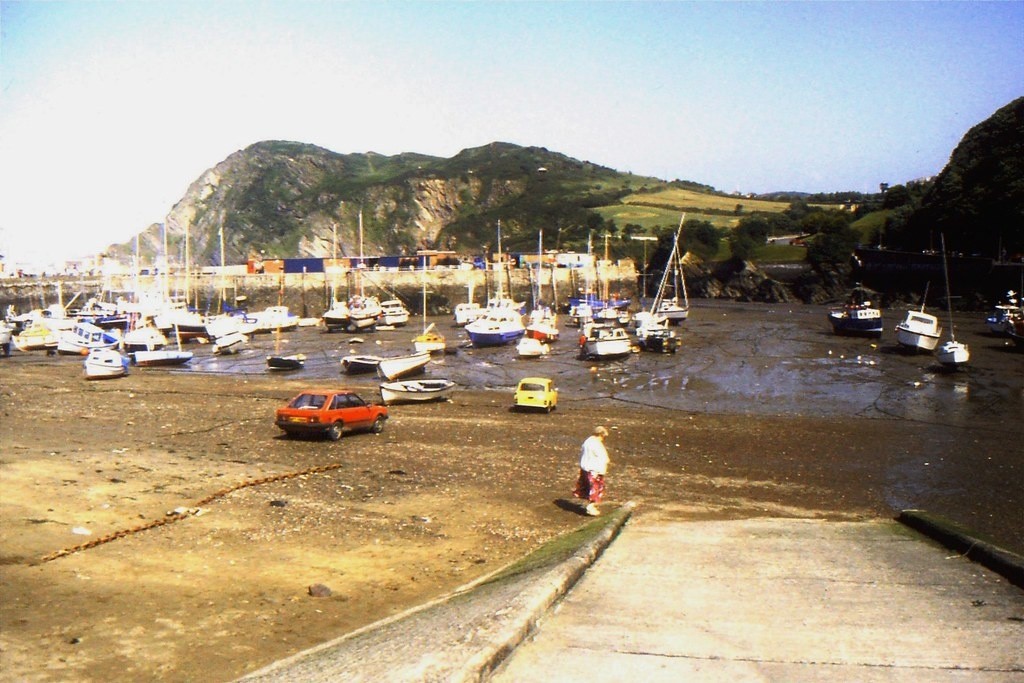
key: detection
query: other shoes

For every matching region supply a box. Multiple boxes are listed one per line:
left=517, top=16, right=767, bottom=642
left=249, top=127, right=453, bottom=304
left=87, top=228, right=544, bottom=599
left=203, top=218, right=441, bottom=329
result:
left=585, top=504, right=601, bottom=516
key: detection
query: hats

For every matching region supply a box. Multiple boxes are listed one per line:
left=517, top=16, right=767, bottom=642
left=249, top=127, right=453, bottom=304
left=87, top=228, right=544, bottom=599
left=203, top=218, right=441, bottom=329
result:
left=592, top=425, right=610, bottom=436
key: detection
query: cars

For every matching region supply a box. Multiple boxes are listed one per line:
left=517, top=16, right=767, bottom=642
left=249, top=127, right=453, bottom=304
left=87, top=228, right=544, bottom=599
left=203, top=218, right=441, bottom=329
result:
left=275, top=389, right=389, bottom=441
left=789, top=236, right=808, bottom=246
left=514, top=378, right=558, bottom=413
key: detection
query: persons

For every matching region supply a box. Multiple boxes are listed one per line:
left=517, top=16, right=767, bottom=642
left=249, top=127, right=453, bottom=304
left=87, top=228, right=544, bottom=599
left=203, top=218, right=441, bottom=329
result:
left=573, top=426, right=610, bottom=515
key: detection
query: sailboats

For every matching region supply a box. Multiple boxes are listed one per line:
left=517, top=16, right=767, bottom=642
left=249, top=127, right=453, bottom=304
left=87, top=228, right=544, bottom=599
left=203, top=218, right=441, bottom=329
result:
left=350, top=213, right=381, bottom=327
left=938, top=233, right=969, bottom=368
left=454, top=220, right=526, bottom=344
left=526, top=229, right=559, bottom=340
left=322, top=224, right=349, bottom=329
left=565, top=234, right=632, bottom=355
left=631, top=212, right=690, bottom=352
left=5, top=218, right=298, bottom=352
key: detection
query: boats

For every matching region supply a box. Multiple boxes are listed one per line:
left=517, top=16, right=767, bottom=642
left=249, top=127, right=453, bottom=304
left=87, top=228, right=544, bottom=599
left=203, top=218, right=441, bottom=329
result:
left=411, top=323, right=446, bottom=350
left=58, top=322, right=119, bottom=354
left=986, top=290, right=1024, bottom=347
left=894, top=305, right=942, bottom=350
left=135, top=351, right=193, bottom=366
left=86, top=348, right=125, bottom=379
left=379, top=379, right=456, bottom=406
left=378, top=300, right=408, bottom=325
left=341, top=355, right=383, bottom=373
left=267, top=354, right=306, bottom=370
left=380, top=351, right=431, bottom=380
left=829, top=301, right=883, bottom=338
